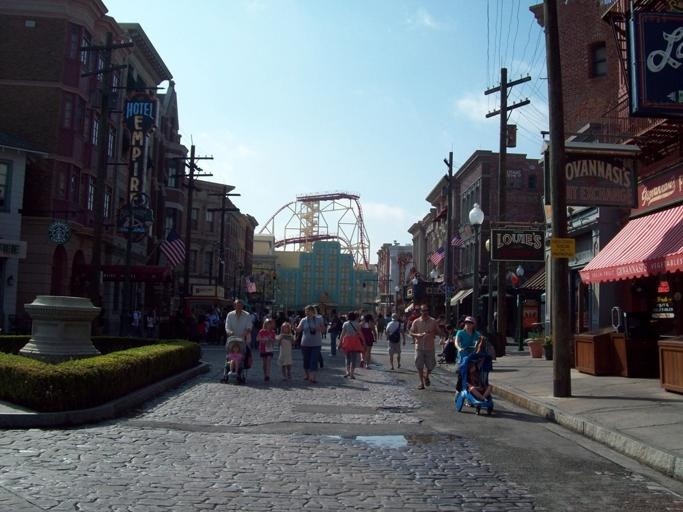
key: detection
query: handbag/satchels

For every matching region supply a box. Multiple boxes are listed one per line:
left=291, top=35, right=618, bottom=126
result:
left=389, top=322, right=400, bottom=344
left=244, top=345, right=252, bottom=369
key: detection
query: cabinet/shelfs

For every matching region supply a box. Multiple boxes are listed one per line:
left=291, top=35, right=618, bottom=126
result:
left=657, top=335, right=682, bottom=393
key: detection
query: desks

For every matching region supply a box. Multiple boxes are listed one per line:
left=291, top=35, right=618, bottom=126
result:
left=574, top=327, right=628, bottom=377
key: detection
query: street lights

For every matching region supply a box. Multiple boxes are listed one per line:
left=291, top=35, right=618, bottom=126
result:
left=393, top=286, right=400, bottom=313
left=429, top=267, right=438, bottom=318
left=469, top=202, right=485, bottom=316
left=513, top=264, right=527, bottom=351
left=411, top=276, right=418, bottom=311
left=210, top=238, right=225, bottom=306
left=484, top=237, right=495, bottom=336
left=120, top=193, right=154, bottom=336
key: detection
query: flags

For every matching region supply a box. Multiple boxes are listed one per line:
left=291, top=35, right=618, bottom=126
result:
left=428, top=247, right=446, bottom=265
left=244, top=275, right=257, bottom=294
left=157, top=228, right=186, bottom=268
left=447, top=230, right=463, bottom=249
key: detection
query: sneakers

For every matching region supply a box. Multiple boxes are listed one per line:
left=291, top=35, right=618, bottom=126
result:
left=417, top=384, right=424, bottom=389
left=425, top=378, right=430, bottom=386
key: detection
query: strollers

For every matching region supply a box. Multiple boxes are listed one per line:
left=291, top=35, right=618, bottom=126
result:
left=453, top=346, right=494, bottom=415
left=221, top=332, right=247, bottom=384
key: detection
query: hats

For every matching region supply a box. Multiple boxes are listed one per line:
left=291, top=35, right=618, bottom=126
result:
left=465, top=317, right=475, bottom=324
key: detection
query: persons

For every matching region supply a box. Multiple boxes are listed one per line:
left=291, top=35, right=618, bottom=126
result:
left=224, top=301, right=250, bottom=342
left=337, top=312, right=365, bottom=379
left=125, top=302, right=497, bottom=364
left=467, top=356, right=491, bottom=401
left=255, top=318, right=287, bottom=383
left=384, top=312, right=405, bottom=370
left=225, top=344, right=244, bottom=376
left=409, top=303, right=440, bottom=389
left=294, top=305, right=325, bottom=383
left=279, top=321, right=297, bottom=380
left=359, top=315, right=377, bottom=369
left=454, top=316, right=483, bottom=407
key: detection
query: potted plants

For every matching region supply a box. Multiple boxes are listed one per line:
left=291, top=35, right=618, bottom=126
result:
left=523, top=330, right=545, bottom=358
left=542, top=335, right=554, bottom=360
